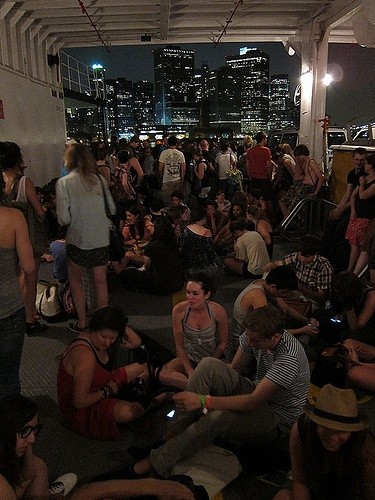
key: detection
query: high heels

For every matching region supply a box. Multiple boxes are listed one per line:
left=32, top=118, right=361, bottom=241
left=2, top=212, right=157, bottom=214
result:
left=26, top=320, right=48, bottom=336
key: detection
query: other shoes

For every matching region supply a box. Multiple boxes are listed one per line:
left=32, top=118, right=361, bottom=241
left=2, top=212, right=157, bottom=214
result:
left=68, top=321, right=86, bottom=333
left=272, top=230, right=279, bottom=238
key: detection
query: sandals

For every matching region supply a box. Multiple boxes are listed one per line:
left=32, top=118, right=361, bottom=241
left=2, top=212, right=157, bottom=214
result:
left=148, top=386, right=176, bottom=409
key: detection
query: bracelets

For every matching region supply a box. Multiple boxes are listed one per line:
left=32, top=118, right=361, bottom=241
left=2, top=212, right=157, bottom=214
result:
left=201, top=395, right=205, bottom=409
left=205, top=394, right=210, bottom=408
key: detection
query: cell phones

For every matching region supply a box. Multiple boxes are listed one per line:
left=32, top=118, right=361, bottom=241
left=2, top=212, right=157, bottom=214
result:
left=164, top=409, right=175, bottom=420
left=329, top=318, right=341, bottom=324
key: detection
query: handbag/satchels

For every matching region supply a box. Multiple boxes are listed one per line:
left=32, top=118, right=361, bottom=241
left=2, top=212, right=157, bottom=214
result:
left=107, top=211, right=125, bottom=260
left=5, top=175, right=48, bottom=256
left=61, top=280, right=76, bottom=314
left=127, top=331, right=173, bottom=367
left=313, top=186, right=325, bottom=198
left=35, top=279, right=62, bottom=318
left=310, top=344, right=349, bottom=390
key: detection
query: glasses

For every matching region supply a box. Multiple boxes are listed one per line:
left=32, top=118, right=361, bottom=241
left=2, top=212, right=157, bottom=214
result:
left=17, top=424, right=41, bottom=439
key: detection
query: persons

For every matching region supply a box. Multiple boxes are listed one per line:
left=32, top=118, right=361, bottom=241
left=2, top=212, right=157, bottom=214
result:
left=0, top=131, right=375, bottom=500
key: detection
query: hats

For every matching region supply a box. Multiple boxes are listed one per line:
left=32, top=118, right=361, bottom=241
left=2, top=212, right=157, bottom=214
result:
left=302, top=384, right=366, bottom=432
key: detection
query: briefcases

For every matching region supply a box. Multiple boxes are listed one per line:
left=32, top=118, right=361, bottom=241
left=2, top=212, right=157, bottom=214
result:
left=281, top=300, right=312, bottom=322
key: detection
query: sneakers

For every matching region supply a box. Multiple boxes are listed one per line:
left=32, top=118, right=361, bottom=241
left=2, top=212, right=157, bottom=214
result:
left=49, top=473, right=78, bottom=497
